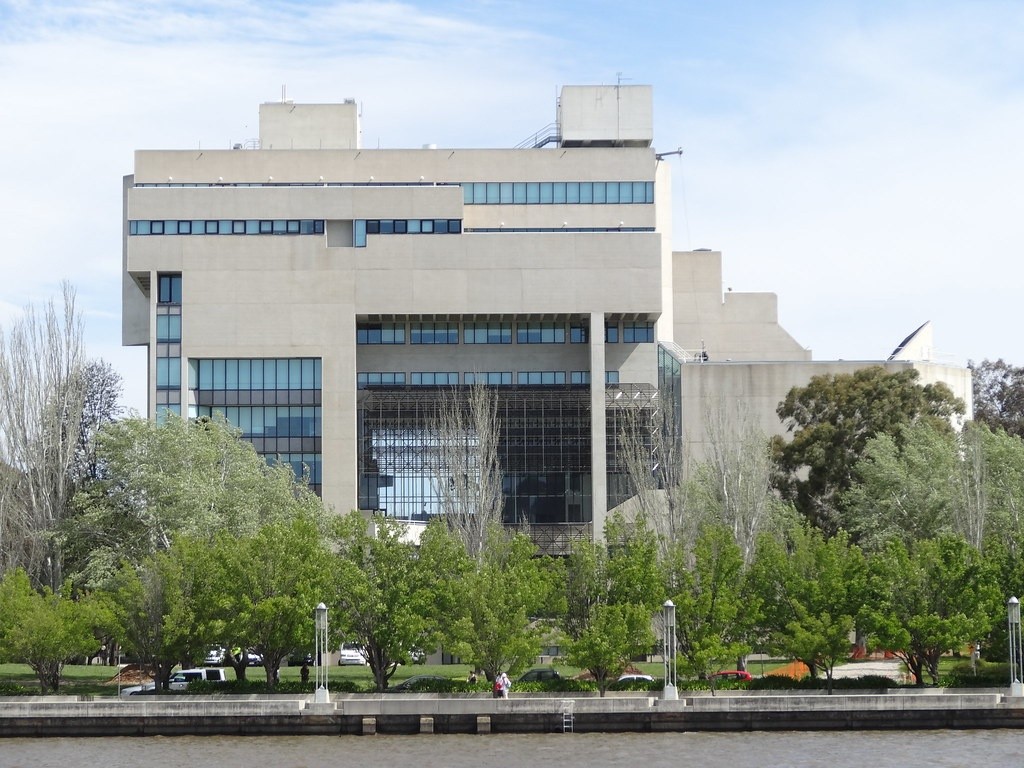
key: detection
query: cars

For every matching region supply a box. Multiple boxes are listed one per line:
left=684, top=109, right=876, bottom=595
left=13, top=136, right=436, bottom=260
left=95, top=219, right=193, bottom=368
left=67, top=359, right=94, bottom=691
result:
left=238, top=646, right=264, bottom=667
left=338, top=641, right=371, bottom=667
left=697, top=671, right=753, bottom=684
left=384, top=675, right=453, bottom=693
left=605, top=675, right=655, bottom=691
left=509, top=668, right=562, bottom=682
left=203, top=644, right=227, bottom=666
left=288, top=649, right=315, bottom=667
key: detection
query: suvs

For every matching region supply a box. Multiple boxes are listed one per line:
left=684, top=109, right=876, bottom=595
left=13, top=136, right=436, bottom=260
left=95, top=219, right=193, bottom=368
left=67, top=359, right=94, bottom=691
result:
left=120, top=666, right=226, bottom=696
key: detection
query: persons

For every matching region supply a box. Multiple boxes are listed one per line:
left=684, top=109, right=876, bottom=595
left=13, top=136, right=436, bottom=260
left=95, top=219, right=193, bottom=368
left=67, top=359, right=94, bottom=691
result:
left=300, top=664, right=309, bottom=682
left=468, top=671, right=476, bottom=684
left=496, top=673, right=511, bottom=699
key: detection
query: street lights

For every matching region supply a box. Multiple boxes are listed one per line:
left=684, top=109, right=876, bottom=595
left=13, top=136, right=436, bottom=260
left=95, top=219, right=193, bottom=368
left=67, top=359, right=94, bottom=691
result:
left=313, top=602, right=331, bottom=703
left=1007, top=596, right=1023, bottom=685
left=661, top=599, right=684, bottom=700
